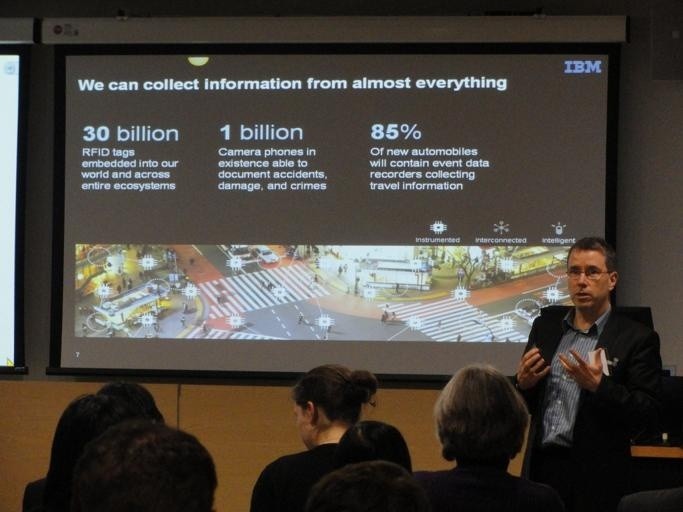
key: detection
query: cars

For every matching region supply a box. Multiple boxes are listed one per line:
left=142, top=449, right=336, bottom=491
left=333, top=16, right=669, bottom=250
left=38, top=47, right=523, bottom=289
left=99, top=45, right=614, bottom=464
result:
left=228, top=243, right=278, bottom=264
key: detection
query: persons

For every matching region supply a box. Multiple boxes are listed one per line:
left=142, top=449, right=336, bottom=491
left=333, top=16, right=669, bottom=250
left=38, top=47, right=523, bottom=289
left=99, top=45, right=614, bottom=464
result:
left=410, top=362, right=565, bottom=511
left=617, top=489, right=683, bottom=512
left=515, top=236, right=667, bottom=511
left=250, top=364, right=431, bottom=511
left=20, top=380, right=218, bottom=511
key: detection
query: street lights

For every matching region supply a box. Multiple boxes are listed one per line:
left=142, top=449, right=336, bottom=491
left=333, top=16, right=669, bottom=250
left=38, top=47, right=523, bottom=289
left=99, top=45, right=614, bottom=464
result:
left=470, top=319, right=496, bottom=342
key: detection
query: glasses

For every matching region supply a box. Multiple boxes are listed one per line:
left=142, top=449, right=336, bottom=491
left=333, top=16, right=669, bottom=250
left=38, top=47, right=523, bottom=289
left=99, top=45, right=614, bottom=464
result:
left=565, top=266, right=613, bottom=280
